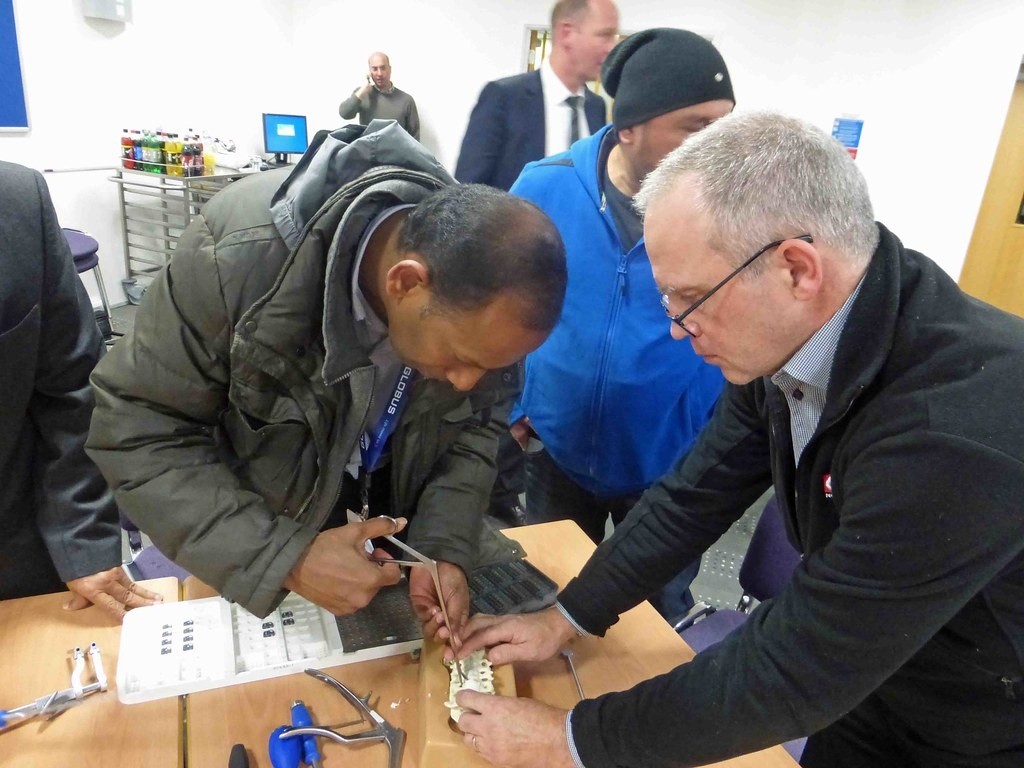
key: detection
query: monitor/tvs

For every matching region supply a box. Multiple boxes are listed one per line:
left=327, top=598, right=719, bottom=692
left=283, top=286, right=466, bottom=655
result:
left=262, top=113, right=308, bottom=165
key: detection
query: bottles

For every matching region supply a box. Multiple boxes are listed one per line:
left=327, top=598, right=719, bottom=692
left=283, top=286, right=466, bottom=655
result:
left=121, top=127, right=237, bottom=177
left=249, top=155, right=267, bottom=172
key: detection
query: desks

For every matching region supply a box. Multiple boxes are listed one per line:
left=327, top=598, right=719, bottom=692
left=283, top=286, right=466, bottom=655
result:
left=0, top=519, right=803, bottom=768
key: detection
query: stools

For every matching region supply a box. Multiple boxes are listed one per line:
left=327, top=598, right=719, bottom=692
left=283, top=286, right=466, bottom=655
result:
left=60, top=229, right=114, bottom=333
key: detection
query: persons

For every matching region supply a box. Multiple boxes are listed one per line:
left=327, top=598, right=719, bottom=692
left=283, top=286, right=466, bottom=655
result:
left=442, top=110, right=1024, bottom=768
left=507, top=28, right=737, bottom=629
left=455, top=0, right=619, bottom=192
left=339, top=52, right=420, bottom=144
left=0, top=162, right=165, bottom=624
left=85, top=119, right=568, bottom=649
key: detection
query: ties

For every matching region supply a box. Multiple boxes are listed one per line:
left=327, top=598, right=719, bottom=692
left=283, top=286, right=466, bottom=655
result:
left=567, top=96, right=580, bottom=147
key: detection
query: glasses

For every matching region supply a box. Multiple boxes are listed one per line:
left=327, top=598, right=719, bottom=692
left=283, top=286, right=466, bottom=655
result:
left=661, top=233, right=814, bottom=337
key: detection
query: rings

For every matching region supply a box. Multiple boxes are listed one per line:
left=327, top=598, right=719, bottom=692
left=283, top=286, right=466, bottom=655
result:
left=473, top=735, right=479, bottom=752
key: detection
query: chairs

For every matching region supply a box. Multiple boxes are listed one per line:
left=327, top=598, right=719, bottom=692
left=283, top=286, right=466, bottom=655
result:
left=668, top=496, right=807, bottom=759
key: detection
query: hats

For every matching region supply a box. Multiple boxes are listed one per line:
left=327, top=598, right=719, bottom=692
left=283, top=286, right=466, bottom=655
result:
left=600, top=27, right=736, bottom=142
